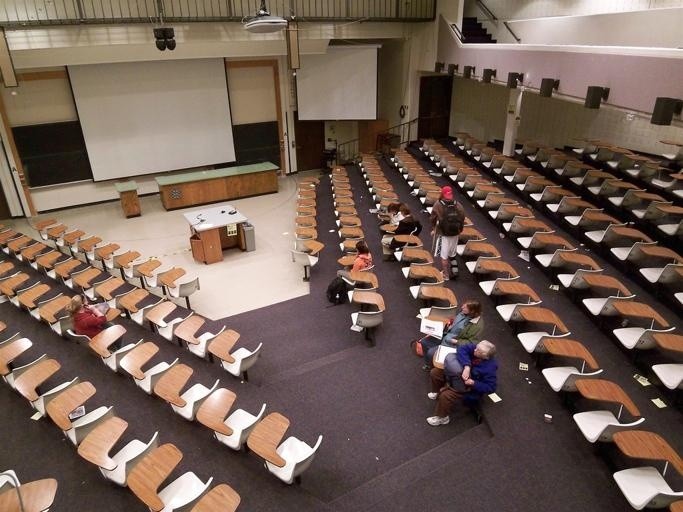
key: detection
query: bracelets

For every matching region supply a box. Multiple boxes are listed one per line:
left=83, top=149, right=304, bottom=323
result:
left=455, top=340, right=458, bottom=344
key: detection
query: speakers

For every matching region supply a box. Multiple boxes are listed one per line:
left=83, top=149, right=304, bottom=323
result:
left=651, top=97, right=683, bottom=125
left=435, top=62, right=444, bottom=73
left=464, top=65, right=475, bottom=79
left=584, top=86, right=610, bottom=109
left=540, top=78, right=560, bottom=97
left=153, top=28, right=176, bottom=50
left=483, top=69, right=496, bottom=83
left=507, top=72, right=523, bottom=89
left=448, top=64, right=458, bottom=76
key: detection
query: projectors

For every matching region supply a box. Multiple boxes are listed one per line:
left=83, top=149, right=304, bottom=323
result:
left=242, top=15, right=288, bottom=33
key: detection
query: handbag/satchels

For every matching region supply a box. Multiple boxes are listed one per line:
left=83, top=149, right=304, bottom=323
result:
left=432, top=345, right=457, bottom=369
left=416, top=342, right=425, bottom=357
left=443, top=353, right=471, bottom=393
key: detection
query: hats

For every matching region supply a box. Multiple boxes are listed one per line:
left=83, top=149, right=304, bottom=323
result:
left=442, top=186, right=453, bottom=200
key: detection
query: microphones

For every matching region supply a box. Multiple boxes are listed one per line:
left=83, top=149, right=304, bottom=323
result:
left=197, top=213, right=206, bottom=222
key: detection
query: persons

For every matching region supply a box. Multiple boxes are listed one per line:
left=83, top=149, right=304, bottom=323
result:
left=430, top=185, right=465, bottom=282
left=336, top=240, right=372, bottom=283
left=65, top=294, right=124, bottom=353
left=427, top=339, right=498, bottom=427
left=419, top=298, right=484, bottom=373
left=380, top=205, right=423, bottom=252
left=388, top=203, right=405, bottom=226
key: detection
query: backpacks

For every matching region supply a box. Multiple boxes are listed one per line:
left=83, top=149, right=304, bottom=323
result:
left=439, top=199, right=464, bottom=236
left=326, top=277, right=347, bottom=305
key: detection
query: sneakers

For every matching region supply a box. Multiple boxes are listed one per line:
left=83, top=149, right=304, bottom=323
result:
left=426, top=416, right=449, bottom=426
left=449, top=272, right=458, bottom=279
left=427, top=392, right=439, bottom=400
